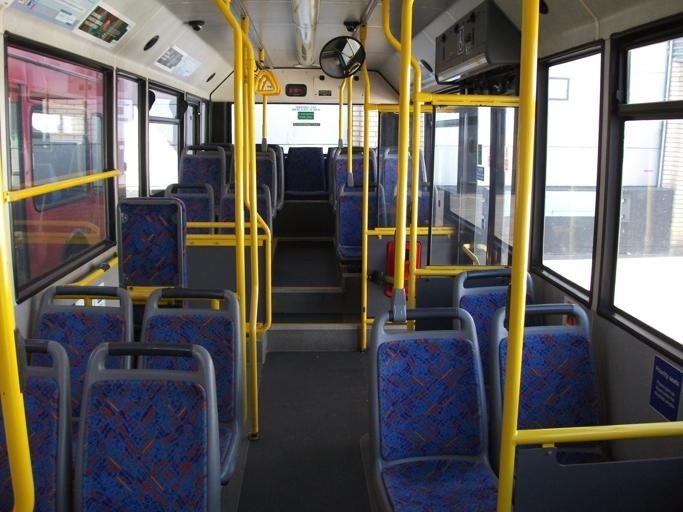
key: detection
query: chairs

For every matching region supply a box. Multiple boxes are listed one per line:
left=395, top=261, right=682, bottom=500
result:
left=137, top=288, right=243, bottom=486
left=110, top=142, right=441, bottom=291
left=484, top=302, right=607, bottom=479
left=3, top=337, right=71, bottom=511
left=74, top=343, right=224, bottom=512
left=27, top=284, right=134, bottom=484
left=455, top=264, right=539, bottom=434
left=369, top=306, right=499, bottom=512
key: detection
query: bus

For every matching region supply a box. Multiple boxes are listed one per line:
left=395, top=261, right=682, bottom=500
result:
left=3, top=47, right=132, bottom=284
